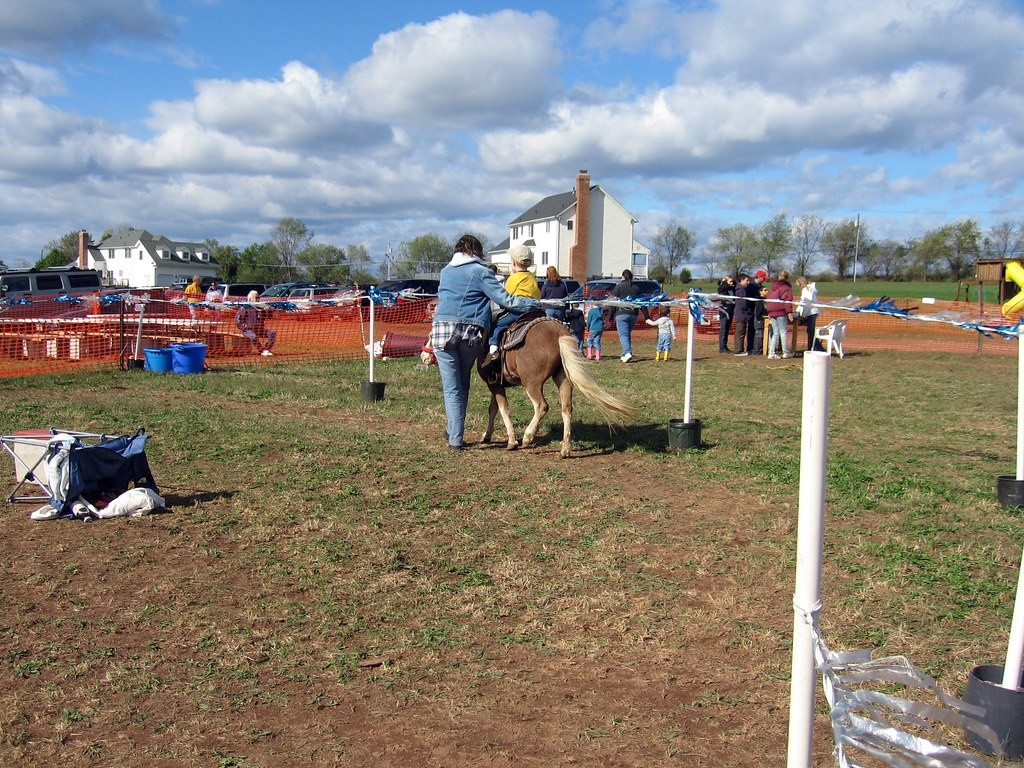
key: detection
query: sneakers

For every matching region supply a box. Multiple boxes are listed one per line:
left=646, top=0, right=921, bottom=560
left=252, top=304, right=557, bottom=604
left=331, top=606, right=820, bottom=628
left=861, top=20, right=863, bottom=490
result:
left=783, top=352, right=795, bottom=359
left=623, top=353, right=632, bottom=362
left=481, top=350, right=499, bottom=368
left=261, top=350, right=273, bottom=356
left=767, top=354, right=782, bottom=360
left=620, top=357, right=625, bottom=360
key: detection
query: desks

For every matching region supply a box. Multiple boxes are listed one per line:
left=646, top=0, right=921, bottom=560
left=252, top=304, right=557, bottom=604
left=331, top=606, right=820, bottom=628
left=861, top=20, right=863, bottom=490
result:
left=762, top=315, right=807, bottom=358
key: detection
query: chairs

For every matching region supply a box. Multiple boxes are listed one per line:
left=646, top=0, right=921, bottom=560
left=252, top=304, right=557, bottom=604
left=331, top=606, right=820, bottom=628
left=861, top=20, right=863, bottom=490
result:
left=811, top=319, right=848, bottom=359
left=0, top=426, right=161, bottom=520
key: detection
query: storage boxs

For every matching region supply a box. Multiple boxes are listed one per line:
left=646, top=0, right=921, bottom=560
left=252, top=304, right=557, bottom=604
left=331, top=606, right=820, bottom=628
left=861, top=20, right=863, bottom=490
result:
left=13, top=429, right=74, bottom=486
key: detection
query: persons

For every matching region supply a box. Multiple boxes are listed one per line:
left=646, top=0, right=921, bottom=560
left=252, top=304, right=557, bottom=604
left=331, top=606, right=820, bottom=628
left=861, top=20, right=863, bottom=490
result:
left=569, top=298, right=587, bottom=354
left=716, top=276, right=737, bottom=354
left=235, top=290, right=277, bottom=356
left=183, top=275, right=202, bottom=319
left=540, top=266, right=567, bottom=322
left=610, top=269, right=649, bottom=363
left=431, top=234, right=543, bottom=448
left=484, top=263, right=506, bottom=324
left=754, top=271, right=795, bottom=359
left=789, top=275, right=827, bottom=352
left=746, top=270, right=770, bottom=356
left=205, top=282, right=223, bottom=304
left=645, top=307, right=677, bottom=362
left=586, top=301, right=603, bottom=362
left=487, top=244, right=541, bottom=354
left=734, top=273, right=756, bottom=357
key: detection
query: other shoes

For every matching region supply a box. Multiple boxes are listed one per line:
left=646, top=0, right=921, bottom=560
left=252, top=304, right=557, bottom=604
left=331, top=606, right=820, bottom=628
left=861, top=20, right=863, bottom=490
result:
left=734, top=351, right=748, bottom=356
left=720, top=349, right=731, bottom=354
left=449, top=445, right=461, bottom=450
left=442, top=431, right=450, bottom=439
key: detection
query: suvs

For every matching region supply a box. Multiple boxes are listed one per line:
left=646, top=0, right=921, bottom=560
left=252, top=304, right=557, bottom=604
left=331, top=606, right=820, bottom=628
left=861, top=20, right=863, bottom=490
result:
left=259, top=281, right=379, bottom=307
left=167, top=275, right=222, bottom=294
left=534, top=275, right=581, bottom=297
left=561, top=277, right=665, bottom=311
left=374, top=278, right=441, bottom=300
left=217, top=282, right=275, bottom=303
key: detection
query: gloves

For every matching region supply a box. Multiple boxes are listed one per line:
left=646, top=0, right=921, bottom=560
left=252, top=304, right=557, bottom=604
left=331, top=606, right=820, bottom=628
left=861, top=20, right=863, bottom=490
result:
left=645, top=315, right=649, bottom=320
left=788, top=313, right=793, bottom=322
left=608, top=317, right=613, bottom=322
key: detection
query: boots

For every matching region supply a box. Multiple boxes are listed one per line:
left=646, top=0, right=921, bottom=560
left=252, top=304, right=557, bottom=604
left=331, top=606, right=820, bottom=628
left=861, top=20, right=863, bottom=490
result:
left=594, top=349, right=600, bottom=361
left=656, top=351, right=661, bottom=361
left=663, top=351, right=669, bottom=361
left=586, top=347, right=592, bottom=360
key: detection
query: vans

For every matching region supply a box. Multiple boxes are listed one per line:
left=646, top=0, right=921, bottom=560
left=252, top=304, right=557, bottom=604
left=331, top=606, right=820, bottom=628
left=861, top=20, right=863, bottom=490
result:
left=0, top=269, right=104, bottom=299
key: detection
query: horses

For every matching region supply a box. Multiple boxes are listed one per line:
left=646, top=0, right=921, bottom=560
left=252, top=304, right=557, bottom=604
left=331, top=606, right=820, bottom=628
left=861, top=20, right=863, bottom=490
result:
left=421, top=304, right=641, bottom=461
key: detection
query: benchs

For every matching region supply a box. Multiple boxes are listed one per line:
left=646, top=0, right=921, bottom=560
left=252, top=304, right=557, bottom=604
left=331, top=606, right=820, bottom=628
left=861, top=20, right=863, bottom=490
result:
left=0, top=332, right=86, bottom=363
left=143, top=328, right=252, bottom=357
left=49, top=330, right=204, bottom=358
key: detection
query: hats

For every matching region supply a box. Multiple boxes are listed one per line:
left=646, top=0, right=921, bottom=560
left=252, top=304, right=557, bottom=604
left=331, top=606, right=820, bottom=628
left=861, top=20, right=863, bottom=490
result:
left=756, top=270, right=770, bottom=279
left=507, top=245, right=532, bottom=266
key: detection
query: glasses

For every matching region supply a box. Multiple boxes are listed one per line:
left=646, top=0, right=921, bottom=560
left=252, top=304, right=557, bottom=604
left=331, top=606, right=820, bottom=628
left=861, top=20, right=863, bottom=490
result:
left=255, top=297, right=261, bottom=299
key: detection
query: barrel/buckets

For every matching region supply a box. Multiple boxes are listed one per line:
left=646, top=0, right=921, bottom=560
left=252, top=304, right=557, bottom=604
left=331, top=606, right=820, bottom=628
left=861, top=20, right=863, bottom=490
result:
left=14, top=428, right=64, bottom=485
left=144, top=348, right=172, bottom=373
left=170, top=343, right=207, bottom=374
